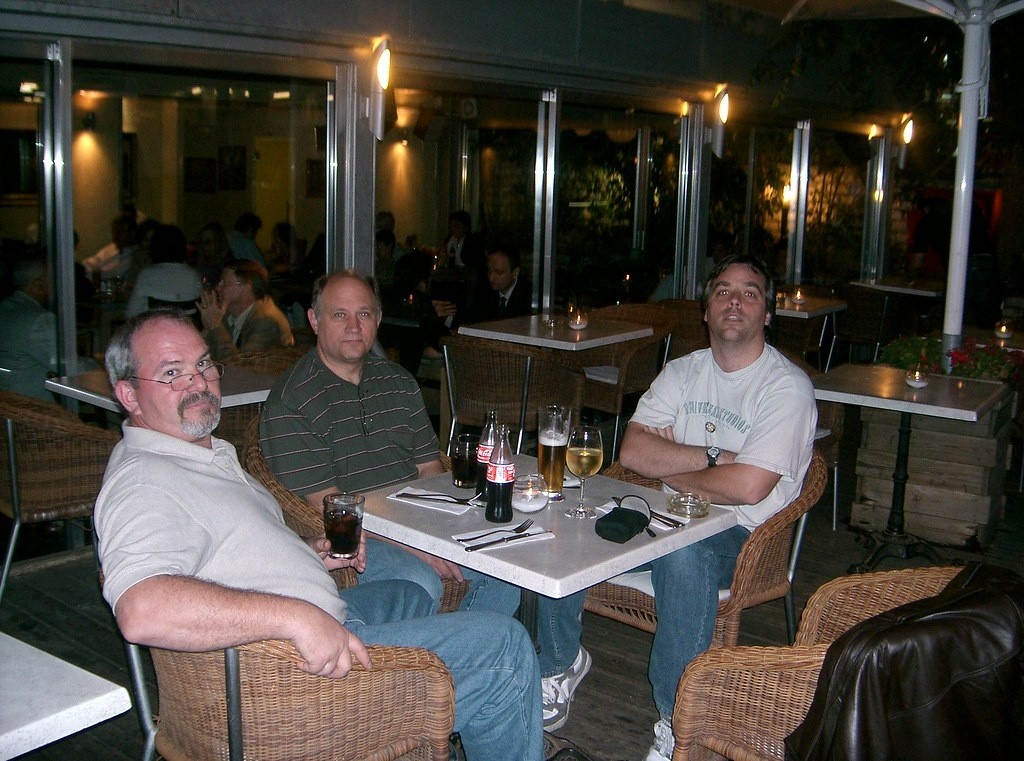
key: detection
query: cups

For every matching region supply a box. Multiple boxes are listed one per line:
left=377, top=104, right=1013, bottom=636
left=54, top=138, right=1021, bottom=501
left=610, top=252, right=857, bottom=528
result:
left=538, top=405, right=571, bottom=500
left=92, top=265, right=101, bottom=284
left=323, top=492, right=366, bottom=559
left=451, top=433, right=480, bottom=488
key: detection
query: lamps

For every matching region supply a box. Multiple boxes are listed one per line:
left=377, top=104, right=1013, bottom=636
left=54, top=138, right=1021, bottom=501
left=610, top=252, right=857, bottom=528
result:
left=87, top=112, right=97, bottom=130
left=897, top=117, right=914, bottom=169
left=712, top=87, right=729, bottom=160
left=366, top=37, right=391, bottom=144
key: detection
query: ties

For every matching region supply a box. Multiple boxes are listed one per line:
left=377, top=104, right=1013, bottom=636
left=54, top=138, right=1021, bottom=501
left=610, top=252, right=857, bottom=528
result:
left=498, top=296, right=507, bottom=318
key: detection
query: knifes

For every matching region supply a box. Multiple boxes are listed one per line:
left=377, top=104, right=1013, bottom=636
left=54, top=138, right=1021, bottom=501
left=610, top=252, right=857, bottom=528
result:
left=396, top=494, right=485, bottom=508
left=465, top=530, right=553, bottom=552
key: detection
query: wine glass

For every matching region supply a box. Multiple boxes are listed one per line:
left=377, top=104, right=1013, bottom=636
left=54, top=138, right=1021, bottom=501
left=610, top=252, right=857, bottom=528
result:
left=566, top=425, right=603, bottom=519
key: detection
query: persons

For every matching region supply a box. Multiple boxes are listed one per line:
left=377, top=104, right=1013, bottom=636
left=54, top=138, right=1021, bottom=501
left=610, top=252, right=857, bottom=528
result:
left=906, top=196, right=999, bottom=329
left=539, top=251, right=818, bottom=761
left=702, top=230, right=999, bottom=330
left=261, top=272, right=521, bottom=616
left=0, top=211, right=307, bottom=546
left=375, top=211, right=531, bottom=376
left=93, top=307, right=589, bottom=761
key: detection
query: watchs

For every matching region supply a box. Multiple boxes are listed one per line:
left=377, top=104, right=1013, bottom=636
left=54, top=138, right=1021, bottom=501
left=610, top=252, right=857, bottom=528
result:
left=705, top=445, right=720, bottom=467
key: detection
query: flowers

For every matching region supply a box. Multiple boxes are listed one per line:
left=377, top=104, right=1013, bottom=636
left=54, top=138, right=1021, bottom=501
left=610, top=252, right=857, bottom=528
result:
left=921, top=333, right=1024, bottom=387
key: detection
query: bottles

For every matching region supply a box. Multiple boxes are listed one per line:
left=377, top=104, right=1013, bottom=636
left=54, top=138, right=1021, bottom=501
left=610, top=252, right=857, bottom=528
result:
left=486, top=424, right=515, bottom=524
left=511, top=474, right=550, bottom=514
left=104, top=270, right=125, bottom=296
left=475, top=410, right=498, bottom=502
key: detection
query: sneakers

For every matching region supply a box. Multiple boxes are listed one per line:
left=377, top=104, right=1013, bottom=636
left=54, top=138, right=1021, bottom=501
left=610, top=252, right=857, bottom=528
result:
left=540, top=645, right=592, bottom=733
left=643, top=718, right=676, bottom=761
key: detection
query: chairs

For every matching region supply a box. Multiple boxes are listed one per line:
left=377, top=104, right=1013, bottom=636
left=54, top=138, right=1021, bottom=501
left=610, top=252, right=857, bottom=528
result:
left=0, top=263, right=1024, bottom=761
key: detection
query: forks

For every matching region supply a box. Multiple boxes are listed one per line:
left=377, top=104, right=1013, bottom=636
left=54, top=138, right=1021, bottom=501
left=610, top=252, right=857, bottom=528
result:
left=400, top=491, right=482, bottom=502
left=457, top=518, right=535, bottom=542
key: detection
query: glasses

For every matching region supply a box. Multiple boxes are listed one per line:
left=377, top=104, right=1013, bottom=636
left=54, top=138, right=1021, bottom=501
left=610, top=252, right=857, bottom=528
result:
left=219, top=279, right=246, bottom=287
left=357, top=384, right=375, bottom=435
left=128, top=361, right=224, bottom=391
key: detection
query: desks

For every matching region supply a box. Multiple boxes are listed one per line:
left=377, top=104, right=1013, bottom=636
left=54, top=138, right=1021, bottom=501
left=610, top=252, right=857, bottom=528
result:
left=774, top=290, right=848, bottom=361
left=809, top=363, right=1012, bottom=576
left=849, top=276, right=944, bottom=344
left=0, top=631, right=133, bottom=761
left=42, top=361, right=275, bottom=437
left=457, top=314, right=654, bottom=356
left=351, top=451, right=737, bottom=761
left=379, top=298, right=435, bottom=328
